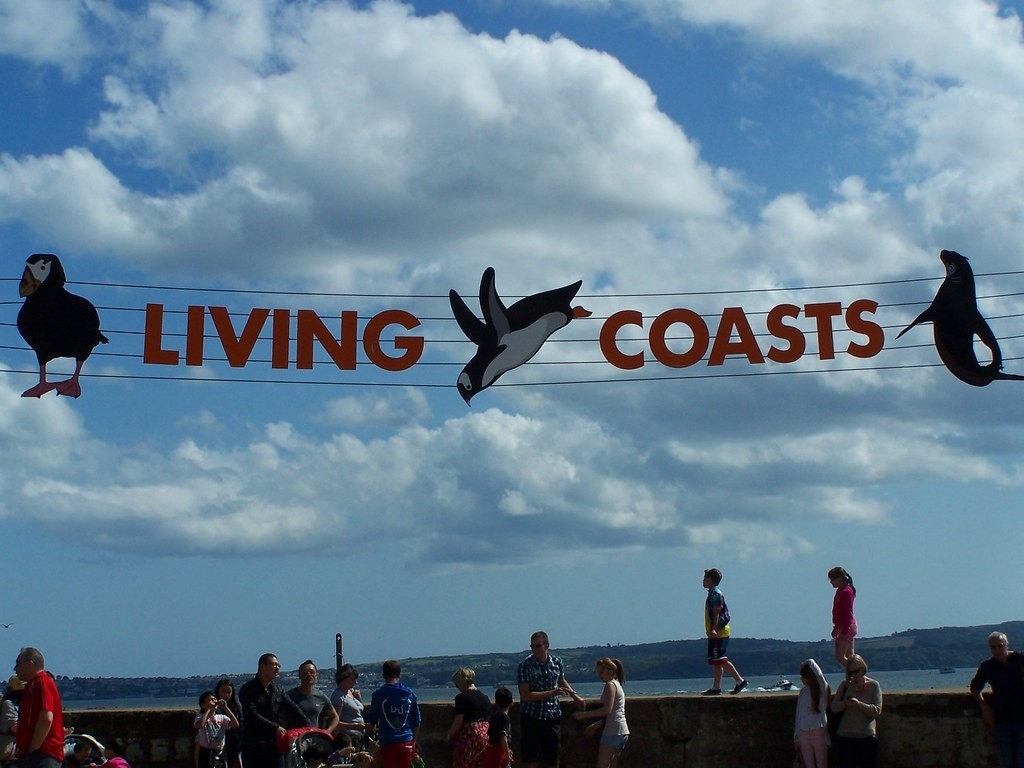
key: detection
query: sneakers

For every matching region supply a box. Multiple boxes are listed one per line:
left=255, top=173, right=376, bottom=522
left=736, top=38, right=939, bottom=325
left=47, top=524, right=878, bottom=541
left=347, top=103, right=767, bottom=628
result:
left=730, top=680, right=749, bottom=694
left=701, top=688, right=722, bottom=695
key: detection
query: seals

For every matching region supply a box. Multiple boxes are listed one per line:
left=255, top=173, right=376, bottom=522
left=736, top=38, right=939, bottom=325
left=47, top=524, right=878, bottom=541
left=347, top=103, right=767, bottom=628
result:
left=894, top=248, right=1024, bottom=387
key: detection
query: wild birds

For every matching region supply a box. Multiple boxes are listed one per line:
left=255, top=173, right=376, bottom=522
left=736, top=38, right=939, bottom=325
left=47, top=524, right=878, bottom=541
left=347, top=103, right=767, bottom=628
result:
left=16, top=253, right=110, bottom=399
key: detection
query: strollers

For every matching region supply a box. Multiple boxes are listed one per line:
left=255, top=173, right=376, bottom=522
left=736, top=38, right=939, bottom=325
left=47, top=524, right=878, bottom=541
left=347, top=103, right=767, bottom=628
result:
left=280, top=722, right=424, bottom=768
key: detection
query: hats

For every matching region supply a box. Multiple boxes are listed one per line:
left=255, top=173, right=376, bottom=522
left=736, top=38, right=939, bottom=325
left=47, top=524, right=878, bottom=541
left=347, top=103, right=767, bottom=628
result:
left=8, top=674, right=27, bottom=691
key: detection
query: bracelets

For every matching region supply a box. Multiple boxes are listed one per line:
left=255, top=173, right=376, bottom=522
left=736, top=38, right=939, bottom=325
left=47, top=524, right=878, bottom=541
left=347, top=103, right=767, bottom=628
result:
left=570, top=691, right=577, bottom=696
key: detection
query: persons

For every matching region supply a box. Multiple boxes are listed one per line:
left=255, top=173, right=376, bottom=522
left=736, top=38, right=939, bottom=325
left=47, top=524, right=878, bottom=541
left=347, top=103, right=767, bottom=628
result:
left=517, top=631, right=586, bottom=768
left=832, top=654, right=883, bottom=768
left=793, top=659, right=831, bottom=768
left=0, top=671, right=57, bottom=768
left=572, top=657, right=630, bottom=768
left=367, top=661, right=422, bottom=768
left=446, top=667, right=493, bottom=768
left=701, top=569, right=748, bottom=695
left=9, top=648, right=63, bottom=768
left=320, top=722, right=425, bottom=768
left=281, top=660, right=339, bottom=768
left=829, top=567, right=868, bottom=679
left=193, top=678, right=242, bottom=768
left=970, top=632, right=1024, bottom=768
left=238, top=654, right=312, bottom=768
left=61, top=739, right=132, bottom=768
left=482, top=687, right=513, bottom=768
left=330, top=664, right=366, bottom=747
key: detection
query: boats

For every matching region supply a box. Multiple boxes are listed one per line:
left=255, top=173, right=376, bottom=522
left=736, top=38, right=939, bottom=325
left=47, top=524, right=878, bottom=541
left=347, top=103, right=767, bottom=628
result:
left=772, top=679, right=794, bottom=690
left=940, top=666, right=955, bottom=674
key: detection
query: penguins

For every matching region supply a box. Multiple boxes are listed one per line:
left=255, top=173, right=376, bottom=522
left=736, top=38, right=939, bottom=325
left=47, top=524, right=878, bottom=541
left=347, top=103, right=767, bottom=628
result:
left=449, top=267, right=592, bottom=407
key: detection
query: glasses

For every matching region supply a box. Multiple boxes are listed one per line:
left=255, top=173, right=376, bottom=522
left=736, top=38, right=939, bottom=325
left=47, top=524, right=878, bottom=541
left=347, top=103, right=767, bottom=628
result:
left=530, top=643, right=546, bottom=648
left=849, top=667, right=862, bottom=676
left=265, top=662, right=280, bottom=668
